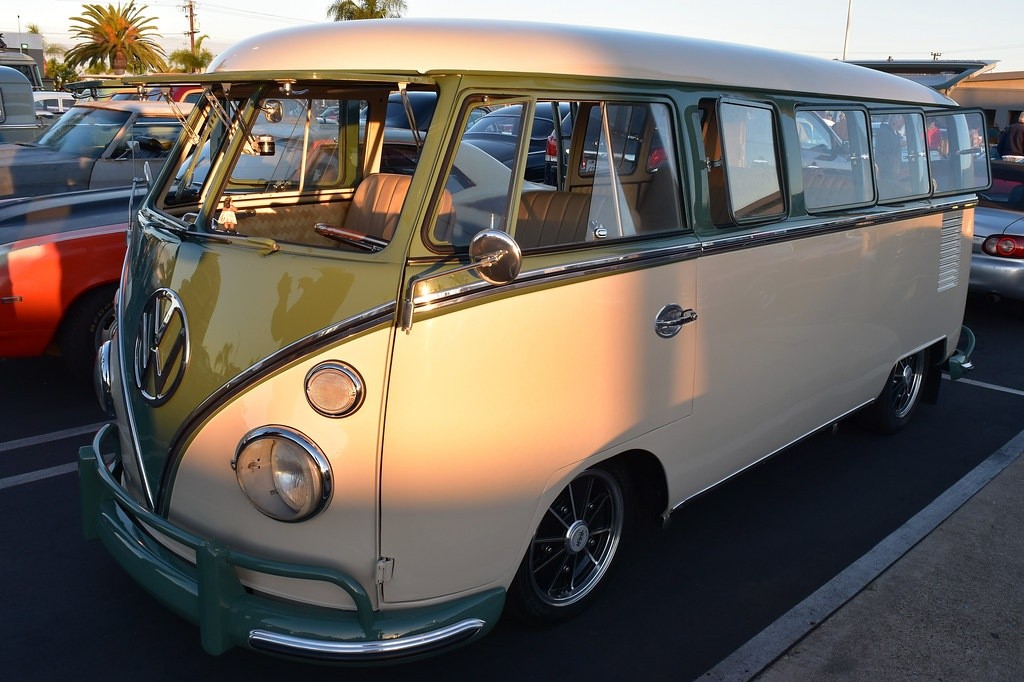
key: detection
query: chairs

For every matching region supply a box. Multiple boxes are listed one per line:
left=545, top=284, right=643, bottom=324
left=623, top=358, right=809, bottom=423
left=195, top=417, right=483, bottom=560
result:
left=125, top=136, right=169, bottom=159
left=495, top=190, right=642, bottom=250
left=334, top=172, right=459, bottom=254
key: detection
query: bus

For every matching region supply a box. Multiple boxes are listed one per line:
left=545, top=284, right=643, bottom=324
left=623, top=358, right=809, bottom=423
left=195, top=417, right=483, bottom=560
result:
left=76, top=15, right=993, bottom=672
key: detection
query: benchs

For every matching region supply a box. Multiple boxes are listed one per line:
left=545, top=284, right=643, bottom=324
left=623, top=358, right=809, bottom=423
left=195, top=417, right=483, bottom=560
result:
left=635, top=164, right=915, bottom=236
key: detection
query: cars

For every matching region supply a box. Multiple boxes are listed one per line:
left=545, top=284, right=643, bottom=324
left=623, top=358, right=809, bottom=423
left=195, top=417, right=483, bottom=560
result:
left=0, top=51, right=1023, bottom=394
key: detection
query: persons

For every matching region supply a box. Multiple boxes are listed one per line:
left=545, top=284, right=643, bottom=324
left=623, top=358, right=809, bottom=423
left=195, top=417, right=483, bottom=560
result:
left=998, top=110, right=1024, bottom=156
left=831, top=109, right=982, bottom=179
